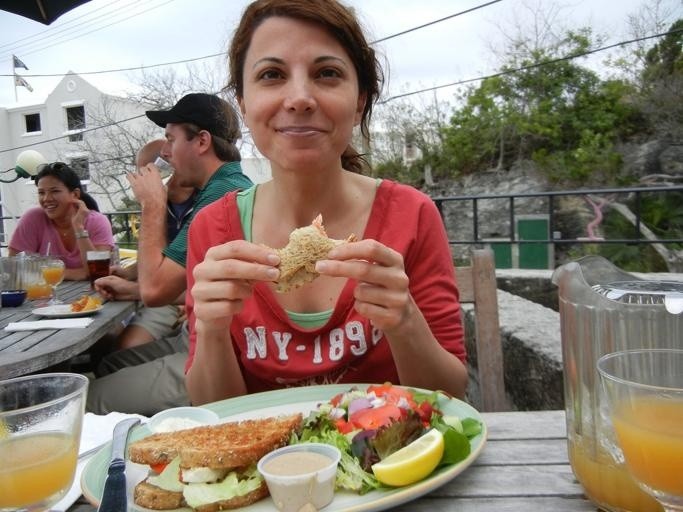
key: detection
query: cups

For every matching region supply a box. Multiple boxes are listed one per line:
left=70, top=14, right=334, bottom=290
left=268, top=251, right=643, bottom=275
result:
left=592, top=344, right=680, bottom=512
left=153, top=157, right=176, bottom=186
left=0, top=372, right=89, bottom=511
left=255, top=442, right=340, bottom=511
left=86, top=249, right=110, bottom=291
left=144, top=400, right=221, bottom=435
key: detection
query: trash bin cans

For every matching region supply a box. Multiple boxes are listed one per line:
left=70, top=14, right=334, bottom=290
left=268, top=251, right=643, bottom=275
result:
left=480, top=215, right=553, bottom=269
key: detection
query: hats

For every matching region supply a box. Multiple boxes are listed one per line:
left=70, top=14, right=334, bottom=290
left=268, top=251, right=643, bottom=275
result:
left=137, top=140, right=166, bottom=175
left=146, top=94, right=239, bottom=145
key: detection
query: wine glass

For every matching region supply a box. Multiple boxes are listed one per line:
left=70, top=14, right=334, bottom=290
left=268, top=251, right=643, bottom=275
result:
left=40, top=259, right=67, bottom=305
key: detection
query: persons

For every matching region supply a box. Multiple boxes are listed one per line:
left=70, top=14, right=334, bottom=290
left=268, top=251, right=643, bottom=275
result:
left=8, top=162, right=116, bottom=281
left=183, top=0, right=468, bottom=406
left=108, top=139, right=199, bottom=350
left=85, top=92, right=254, bottom=418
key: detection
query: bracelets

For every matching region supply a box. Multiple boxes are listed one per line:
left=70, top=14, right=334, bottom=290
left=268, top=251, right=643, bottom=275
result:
left=75, top=230, right=89, bottom=239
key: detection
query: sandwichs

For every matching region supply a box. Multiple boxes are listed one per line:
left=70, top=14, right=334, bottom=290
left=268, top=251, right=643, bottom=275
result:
left=127, top=410, right=304, bottom=511
left=256, top=212, right=359, bottom=294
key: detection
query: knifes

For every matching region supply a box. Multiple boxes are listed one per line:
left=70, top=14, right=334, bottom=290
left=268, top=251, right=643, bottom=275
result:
left=97, top=415, right=143, bottom=512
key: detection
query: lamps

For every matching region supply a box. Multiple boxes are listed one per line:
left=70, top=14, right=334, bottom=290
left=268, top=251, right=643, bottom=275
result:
left=0, top=150, right=48, bottom=183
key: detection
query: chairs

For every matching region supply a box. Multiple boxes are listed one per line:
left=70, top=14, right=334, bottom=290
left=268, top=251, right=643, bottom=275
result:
left=453, top=248, right=508, bottom=412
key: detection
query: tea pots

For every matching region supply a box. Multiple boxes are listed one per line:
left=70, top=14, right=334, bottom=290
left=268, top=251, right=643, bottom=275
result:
left=18, top=254, right=49, bottom=301
left=0, top=255, right=24, bottom=308
left=544, top=254, right=682, bottom=512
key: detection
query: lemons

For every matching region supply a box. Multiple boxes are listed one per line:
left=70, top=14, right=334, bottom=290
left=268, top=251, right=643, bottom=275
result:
left=370, top=426, right=445, bottom=487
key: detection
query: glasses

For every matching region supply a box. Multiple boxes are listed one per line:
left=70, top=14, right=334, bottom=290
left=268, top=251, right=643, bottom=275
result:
left=37, top=163, right=64, bottom=174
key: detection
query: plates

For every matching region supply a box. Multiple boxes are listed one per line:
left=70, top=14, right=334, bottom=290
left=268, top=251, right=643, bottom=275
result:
left=78, top=382, right=488, bottom=512
left=30, top=304, right=103, bottom=317
